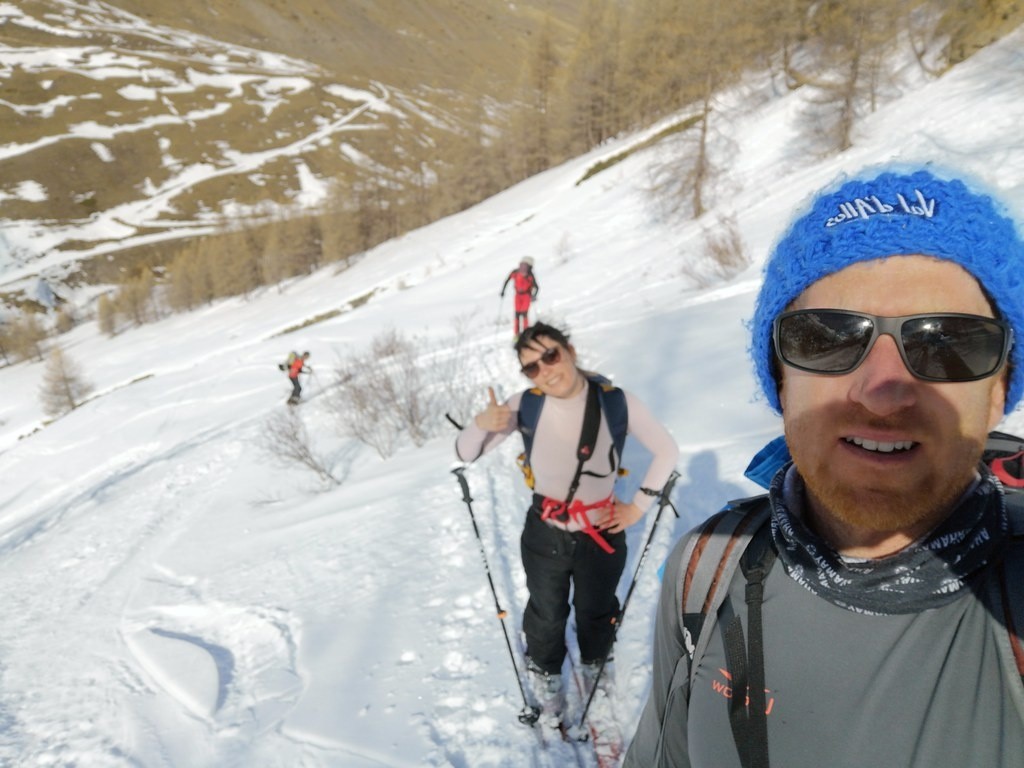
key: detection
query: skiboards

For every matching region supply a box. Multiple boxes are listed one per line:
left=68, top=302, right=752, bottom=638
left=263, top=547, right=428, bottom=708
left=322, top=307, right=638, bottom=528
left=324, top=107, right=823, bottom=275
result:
left=515, top=610, right=627, bottom=767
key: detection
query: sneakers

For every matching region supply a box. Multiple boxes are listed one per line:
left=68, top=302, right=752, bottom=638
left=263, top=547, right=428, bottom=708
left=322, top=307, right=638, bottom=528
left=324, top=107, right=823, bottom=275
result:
left=523, top=654, right=567, bottom=714
left=583, top=652, right=617, bottom=704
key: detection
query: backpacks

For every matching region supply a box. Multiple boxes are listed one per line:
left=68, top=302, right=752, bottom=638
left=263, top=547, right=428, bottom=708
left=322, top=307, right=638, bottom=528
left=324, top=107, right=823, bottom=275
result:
left=288, top=352, right=297, bottom=368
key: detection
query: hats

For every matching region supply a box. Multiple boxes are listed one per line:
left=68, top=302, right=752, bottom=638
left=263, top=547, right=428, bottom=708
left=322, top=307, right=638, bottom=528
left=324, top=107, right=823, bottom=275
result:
left=751, top=160, right=1024, bottom=423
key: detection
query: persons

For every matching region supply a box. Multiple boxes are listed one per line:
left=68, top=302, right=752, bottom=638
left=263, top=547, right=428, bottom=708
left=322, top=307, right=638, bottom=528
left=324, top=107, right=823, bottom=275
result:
left=500, top=257, right=538, bottom=336
left=618, top=163, right=1024, bottom=768
left=284, top=350, right=311, bottom=406
left=454, top=323, right=682, bottom=726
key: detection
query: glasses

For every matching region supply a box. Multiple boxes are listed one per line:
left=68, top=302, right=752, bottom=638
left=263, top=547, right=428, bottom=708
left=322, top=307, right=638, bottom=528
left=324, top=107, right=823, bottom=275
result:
left=520, top=341, right=563, bottom=378
left=770, top=308, right=1014, bottom=382
